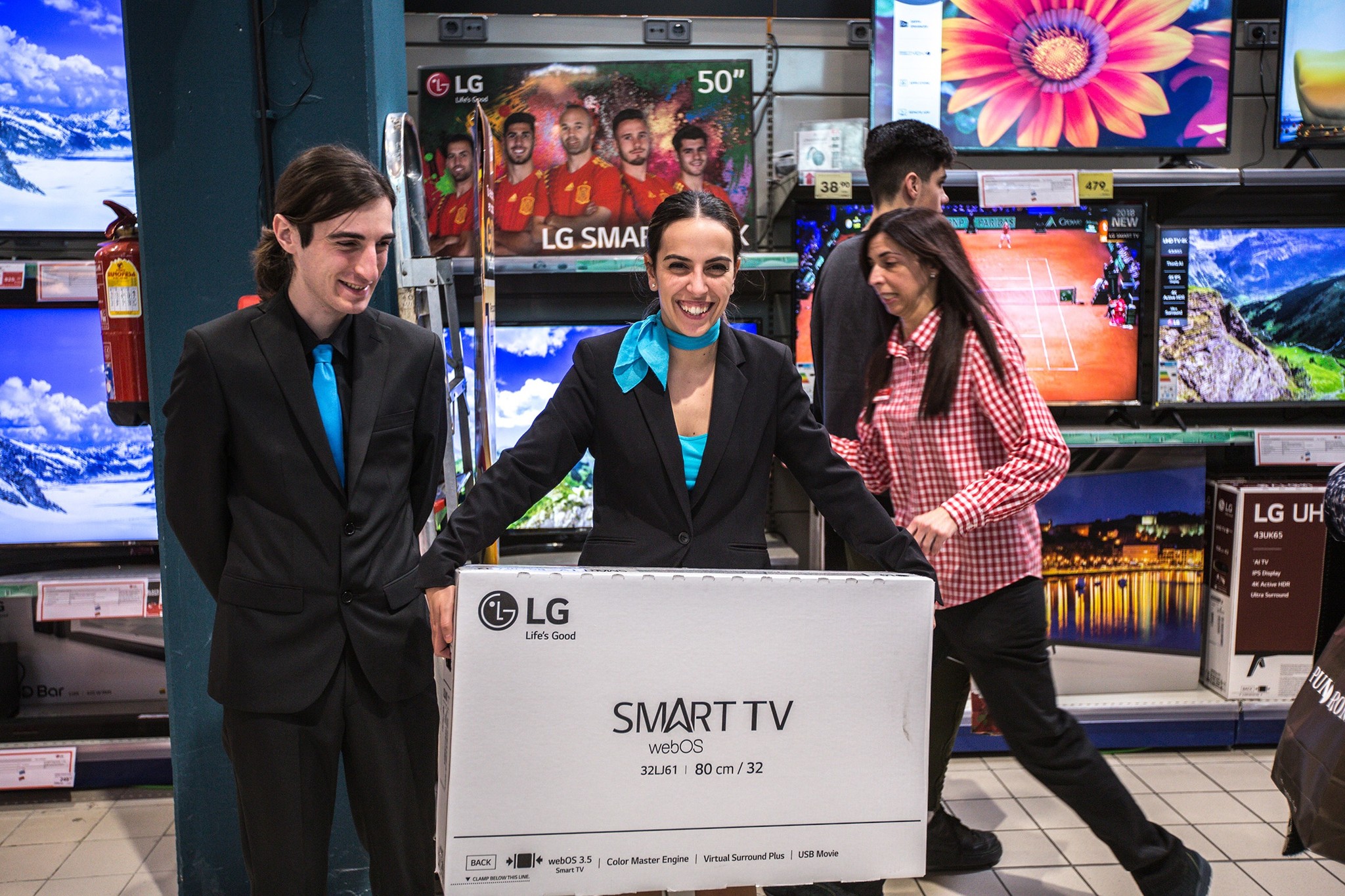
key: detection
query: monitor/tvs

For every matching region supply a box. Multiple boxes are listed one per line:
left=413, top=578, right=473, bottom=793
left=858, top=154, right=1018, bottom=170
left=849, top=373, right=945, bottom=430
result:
left=789, top=199, right=1148, bottom=409
left=417, top=58, right=772, bottom=258
left=442, top=316, right=764, bottom=543
left=0, top=0, right=141, bottom=238
left=1150, top=223, right=1345, bottom=409
left=867, top=0, right=1236, bottom=157
left=0, top=303, right=159, bottom=550
left=1273, top=0, right=1345, bottom=150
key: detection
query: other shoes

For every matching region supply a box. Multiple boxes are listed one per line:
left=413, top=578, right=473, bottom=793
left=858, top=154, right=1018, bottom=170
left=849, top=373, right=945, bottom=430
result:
left=1164, top=846, right=1212, bottom=896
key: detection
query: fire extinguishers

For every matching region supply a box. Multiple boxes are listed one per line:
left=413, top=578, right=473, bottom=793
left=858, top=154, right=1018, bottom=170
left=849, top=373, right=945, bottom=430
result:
left=93, top=199, right=152, bottom=426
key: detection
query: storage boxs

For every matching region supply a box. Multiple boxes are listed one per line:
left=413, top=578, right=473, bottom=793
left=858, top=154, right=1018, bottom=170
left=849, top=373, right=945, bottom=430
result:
left=1198, top=476, right=1330, bottom=702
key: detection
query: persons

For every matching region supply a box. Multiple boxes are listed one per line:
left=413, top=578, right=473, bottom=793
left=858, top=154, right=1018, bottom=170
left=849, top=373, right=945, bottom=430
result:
left=672, top=126, right=750, bottom=253
left=799, top=224, right=830, bottom=276
left=966, top=210, right=977, bottom=234
left=427, top=133, right=475, bottom=257
left=782, top=208, right=1211, bottom=896
left=418, top=190, right=944, bottom=896
left=478, top=112, right=543, bottom=257
left=998, top=222, right=1011, bottom=248
left=1091, top=242, right=1140, bottom=291
left=612, top=109, right=678, bottom=255
left=531, top=105, right=623, bottom=257
left=1107, top=293, right=1127, bottom=331
left=810, top=119, right=1002, bottom=872
left=162, top=145, right=448, bottom=895
left=1035, top=213, right=1046, bottom=231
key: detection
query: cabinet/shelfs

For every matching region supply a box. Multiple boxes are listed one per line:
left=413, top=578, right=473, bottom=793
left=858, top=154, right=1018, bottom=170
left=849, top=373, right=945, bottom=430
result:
left=787, top=150, right=1345, bottom=742
left=385, top=104, right=817, bottom=628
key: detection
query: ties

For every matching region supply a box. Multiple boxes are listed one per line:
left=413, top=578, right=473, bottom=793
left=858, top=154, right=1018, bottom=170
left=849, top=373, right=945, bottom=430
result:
left=310, top=343, right=347, bottom=491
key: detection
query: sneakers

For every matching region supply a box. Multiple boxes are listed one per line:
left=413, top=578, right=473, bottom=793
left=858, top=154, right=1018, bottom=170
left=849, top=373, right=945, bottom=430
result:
left=927, top=810, right=1003, bottom=873
